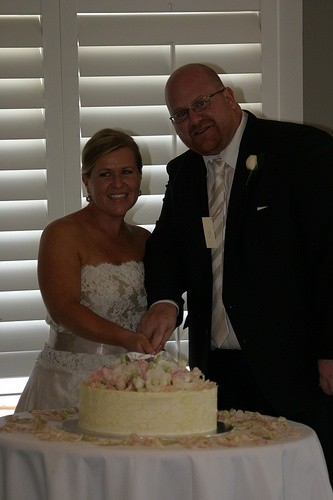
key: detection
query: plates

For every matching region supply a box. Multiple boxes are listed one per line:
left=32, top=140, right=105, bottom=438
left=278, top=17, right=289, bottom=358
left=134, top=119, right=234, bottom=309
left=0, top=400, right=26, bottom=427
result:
left=61, top=421, right=233, bottom=438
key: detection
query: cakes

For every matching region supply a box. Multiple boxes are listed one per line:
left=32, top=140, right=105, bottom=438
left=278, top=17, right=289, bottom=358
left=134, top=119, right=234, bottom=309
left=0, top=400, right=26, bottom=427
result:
left=79, top=352, right=219, bottom=438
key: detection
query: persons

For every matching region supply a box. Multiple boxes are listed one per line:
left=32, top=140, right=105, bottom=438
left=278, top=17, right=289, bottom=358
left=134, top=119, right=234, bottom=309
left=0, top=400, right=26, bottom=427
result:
left=135, top=63, right=333, bottom=483
left=12, top=128, right=152, bottom=414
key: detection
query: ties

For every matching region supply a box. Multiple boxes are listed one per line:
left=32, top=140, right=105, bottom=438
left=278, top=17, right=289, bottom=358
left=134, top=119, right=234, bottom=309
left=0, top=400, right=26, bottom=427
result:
left=208, top=158, right=230, bottom=347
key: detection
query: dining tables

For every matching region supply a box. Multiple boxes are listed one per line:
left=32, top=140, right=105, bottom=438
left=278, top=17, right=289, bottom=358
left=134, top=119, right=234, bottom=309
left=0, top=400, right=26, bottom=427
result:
left=0, top=406, right=333, bottom=500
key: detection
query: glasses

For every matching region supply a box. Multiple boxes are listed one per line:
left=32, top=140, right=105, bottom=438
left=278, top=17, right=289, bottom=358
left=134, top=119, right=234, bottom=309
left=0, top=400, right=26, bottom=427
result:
left=169, top=88, right=225, bottom=122
